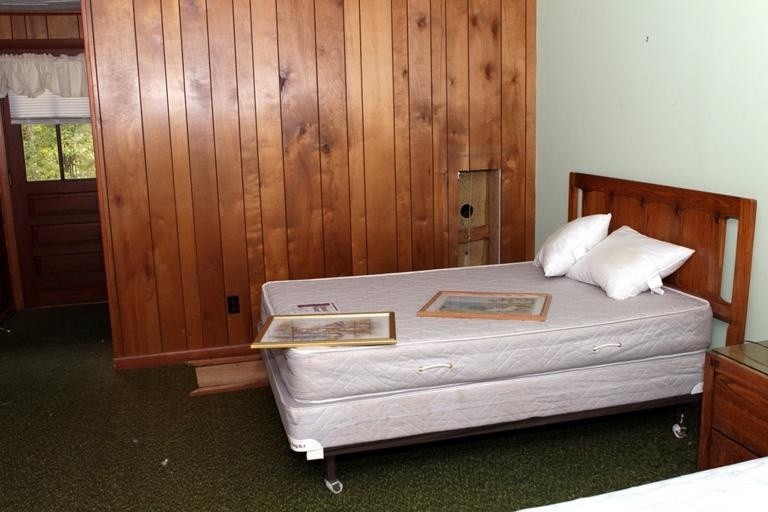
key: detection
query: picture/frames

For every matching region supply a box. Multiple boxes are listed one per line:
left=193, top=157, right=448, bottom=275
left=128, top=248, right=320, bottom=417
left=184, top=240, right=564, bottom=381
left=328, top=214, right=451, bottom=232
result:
left=413, top=290, right=553, bottom=323
left=249, top=309, right=397, bottom=349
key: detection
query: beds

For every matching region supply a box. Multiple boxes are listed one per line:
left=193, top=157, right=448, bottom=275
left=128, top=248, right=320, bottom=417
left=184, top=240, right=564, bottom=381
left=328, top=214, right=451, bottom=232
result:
left=255, top=168, right=760, bottom=495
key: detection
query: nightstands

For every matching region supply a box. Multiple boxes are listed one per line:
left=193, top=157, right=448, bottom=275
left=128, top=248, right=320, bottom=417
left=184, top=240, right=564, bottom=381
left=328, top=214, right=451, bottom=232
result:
left=695, top=339, right=767, bottom=471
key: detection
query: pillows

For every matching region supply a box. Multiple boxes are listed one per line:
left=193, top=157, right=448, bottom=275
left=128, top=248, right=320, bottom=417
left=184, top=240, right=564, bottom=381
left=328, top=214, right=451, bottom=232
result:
left=564, top=222, right=698, bottom=301
left=533, top=210, right=616, bottom=278
